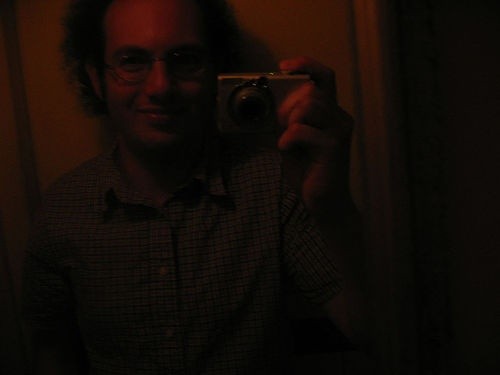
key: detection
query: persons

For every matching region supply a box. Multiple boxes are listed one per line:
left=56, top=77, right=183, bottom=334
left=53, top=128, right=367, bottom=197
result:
left=20, top=0, right=375, bottom=375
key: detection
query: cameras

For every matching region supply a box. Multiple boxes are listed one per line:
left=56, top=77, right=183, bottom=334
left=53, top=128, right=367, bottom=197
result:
left=215, top=72, right=311, bottom=134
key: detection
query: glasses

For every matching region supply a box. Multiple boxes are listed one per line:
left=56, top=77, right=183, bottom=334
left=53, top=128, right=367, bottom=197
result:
left=77, top=44, right=218, bottom=84
